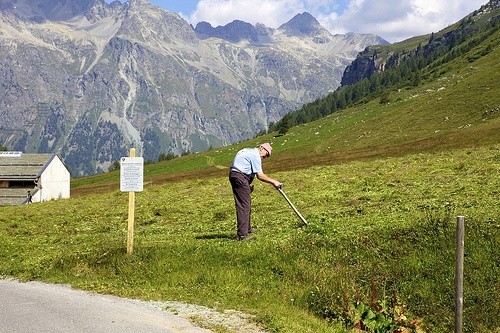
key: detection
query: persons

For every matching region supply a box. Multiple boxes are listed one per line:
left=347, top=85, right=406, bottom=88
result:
left=228, top=141, right=284, bottom=241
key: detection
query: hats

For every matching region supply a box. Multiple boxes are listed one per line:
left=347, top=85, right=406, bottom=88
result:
left=258, top=142, right=273, bottom=158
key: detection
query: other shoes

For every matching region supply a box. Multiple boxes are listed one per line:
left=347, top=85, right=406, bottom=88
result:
left=248, top=228, right=258, bottom=233
left=238, top=236, right=256, bottom=241
